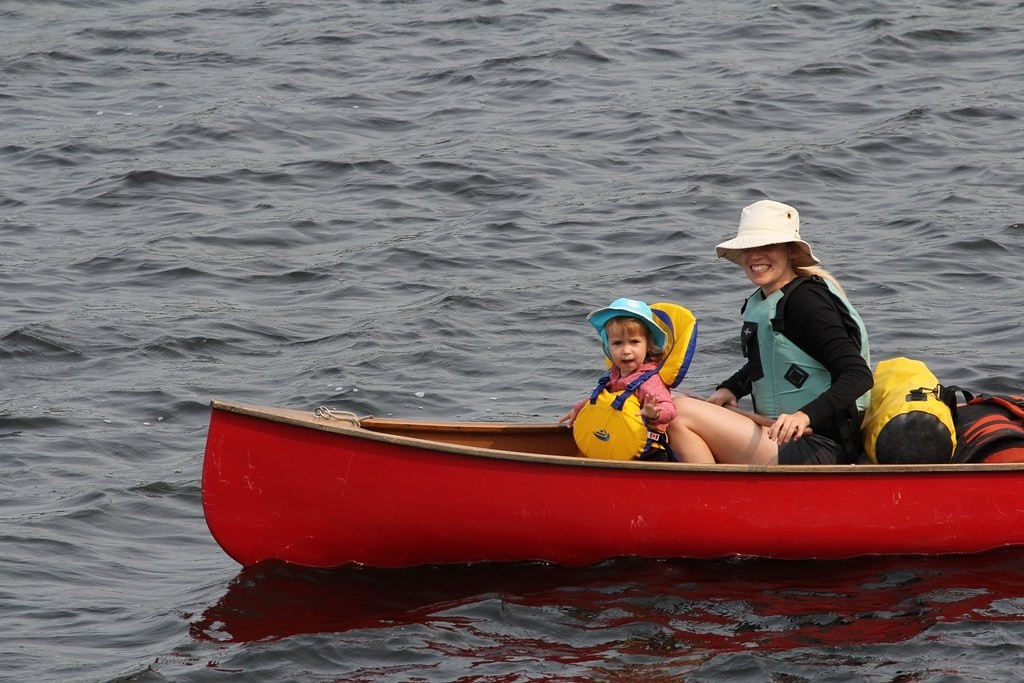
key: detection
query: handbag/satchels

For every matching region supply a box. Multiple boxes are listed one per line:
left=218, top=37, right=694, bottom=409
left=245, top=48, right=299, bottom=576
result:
left=860, top=356, right=959, bottom=464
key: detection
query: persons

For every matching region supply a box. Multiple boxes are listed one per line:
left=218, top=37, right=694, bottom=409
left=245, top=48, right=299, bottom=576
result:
left=559, top=295, right=677, bottom=462
left=658, top=199, right=875, bottom=465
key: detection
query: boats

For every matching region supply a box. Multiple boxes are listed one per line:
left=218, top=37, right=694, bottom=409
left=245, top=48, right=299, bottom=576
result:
left=201, top=399, right=1024, bottom=569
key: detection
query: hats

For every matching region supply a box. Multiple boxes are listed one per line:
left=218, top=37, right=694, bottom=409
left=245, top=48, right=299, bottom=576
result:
left=715, top=199, right=820, bottom=267
left=585, top=298, right=668, bottom=360
